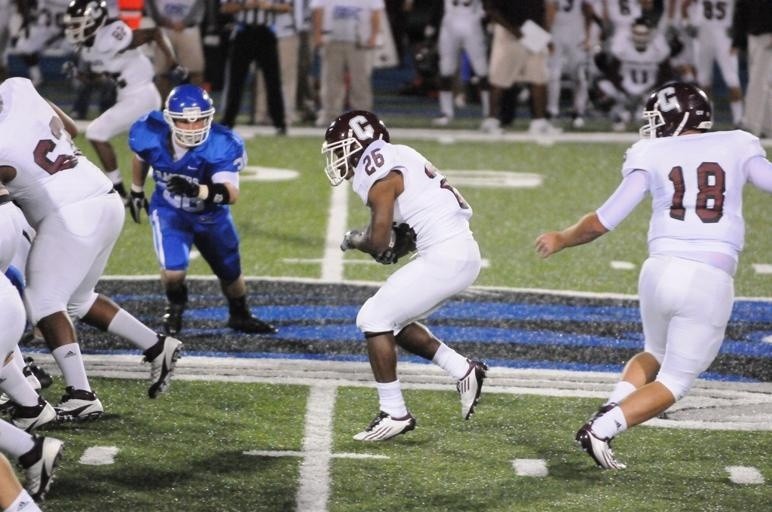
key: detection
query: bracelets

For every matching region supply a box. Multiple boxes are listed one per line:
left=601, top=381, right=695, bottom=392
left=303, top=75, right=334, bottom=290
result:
left=197, top=184, right=210, bottom=201
left=130, top=183, right=145, bottom=193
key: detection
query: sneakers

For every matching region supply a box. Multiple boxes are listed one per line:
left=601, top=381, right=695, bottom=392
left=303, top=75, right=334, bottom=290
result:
left=574, top=403, right=630, bottom=472
left=161, top=296, right=187, bottom=334
left=226, top=311, right=279, bottom=334
left=20, top=435, right=66, bottom=503
left=373, top=248, right=399, bottom=265
left=455, top=357, right=489, bottom=422
left=432, top=114, right=630, bottom=134
left=352, top=410, right=418, bottom=442
left=0, top=359, right=58, bottom=433
left=18, top=334, right=45, bottom=348
left=246, top=113, right=331, bottom=137
left=144, top=337, right=183, bottom=403
left=340, top=229, right=362, bottom=252
left=44, top=386, right=103, bottom=428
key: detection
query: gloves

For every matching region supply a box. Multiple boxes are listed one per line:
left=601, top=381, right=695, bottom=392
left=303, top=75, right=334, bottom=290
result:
left=129, top=188, right=150, bottom=224
left=164, top=175, right=201, bottom=201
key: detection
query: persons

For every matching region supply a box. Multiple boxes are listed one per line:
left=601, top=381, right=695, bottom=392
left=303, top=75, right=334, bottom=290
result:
left=320, top=109, right=487, bottom=444
left=0, top=75, right=187, bottom=511
left=531, top=79, right=770, bottom=469
left=127, top=84, right=281, bottom=337
left=0, top=0, right=772, bottom=138
left=65, top=0, right=193, bottom=211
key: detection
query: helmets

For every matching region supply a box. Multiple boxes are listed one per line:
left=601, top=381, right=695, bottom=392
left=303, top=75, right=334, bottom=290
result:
left=320, top=109, right=389, bottom=188
left=62, top=0, right=108, bottom=48
left=162, top=83, right=216, bottom=149
left=638, top=80, right=716, bottom=140
left=628, top=18, right=654, bottom=47
